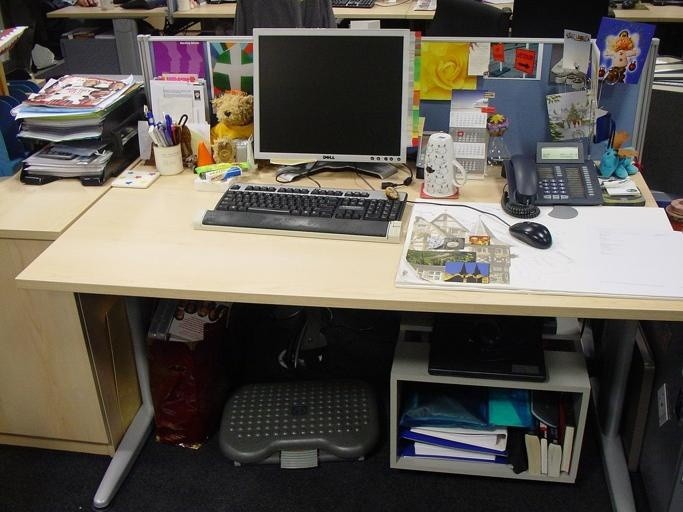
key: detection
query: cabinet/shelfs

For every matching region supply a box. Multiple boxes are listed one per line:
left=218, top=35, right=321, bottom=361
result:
left=1, top=159, right=138, bottom=454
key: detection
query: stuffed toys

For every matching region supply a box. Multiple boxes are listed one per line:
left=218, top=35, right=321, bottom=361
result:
left=211, top=90, right=255, bottom=163
left=598, top=146, right=638, bottom=179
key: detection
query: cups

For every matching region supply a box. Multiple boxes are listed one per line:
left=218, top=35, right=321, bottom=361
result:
left=423, top=132, right=467, bottom=197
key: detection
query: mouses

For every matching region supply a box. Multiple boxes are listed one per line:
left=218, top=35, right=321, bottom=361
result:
left=622, top=0, right=635, bottom=9
left=508, top=221, right=552, bottom=250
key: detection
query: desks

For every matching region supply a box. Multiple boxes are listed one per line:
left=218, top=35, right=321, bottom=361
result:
left=16, top=151, right=682, bottom=510
left=0, top=1, right=680, bottom=151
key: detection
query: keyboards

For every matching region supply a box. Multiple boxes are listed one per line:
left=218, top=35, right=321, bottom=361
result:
left=332, top=0, right=375, bottom=8
left=194, top=181, right=408, bottom=245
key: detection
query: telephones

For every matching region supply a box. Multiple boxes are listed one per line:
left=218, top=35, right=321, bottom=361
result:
left=504, top=142, right=605, bottom=206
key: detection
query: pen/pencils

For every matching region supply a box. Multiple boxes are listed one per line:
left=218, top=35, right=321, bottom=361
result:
left=147, top=110, right=181, bottom=147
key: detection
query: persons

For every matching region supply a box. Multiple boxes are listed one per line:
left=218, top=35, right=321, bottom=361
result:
left=45, top=0, right=96, bottom=47
left=174, top=299, right=225, bottom=321
left=606, top=29, right=641, bottom=82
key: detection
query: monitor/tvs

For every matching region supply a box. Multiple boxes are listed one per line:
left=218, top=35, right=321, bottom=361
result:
left=252, top=26, right=411, bottom=183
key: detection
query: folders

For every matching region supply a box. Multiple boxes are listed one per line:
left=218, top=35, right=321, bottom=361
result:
left=401, top=426, right=510, bottom=465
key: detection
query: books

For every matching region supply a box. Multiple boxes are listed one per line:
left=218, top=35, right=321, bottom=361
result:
left=404, top=397, right=577, bottom=479
left=415, top=0, right=436, bottom=11
left=10, top=70, right=136, bottom=177
left=428, top=313, right=548, bottom=384
left=169, top=307, right=230, bottom=342
